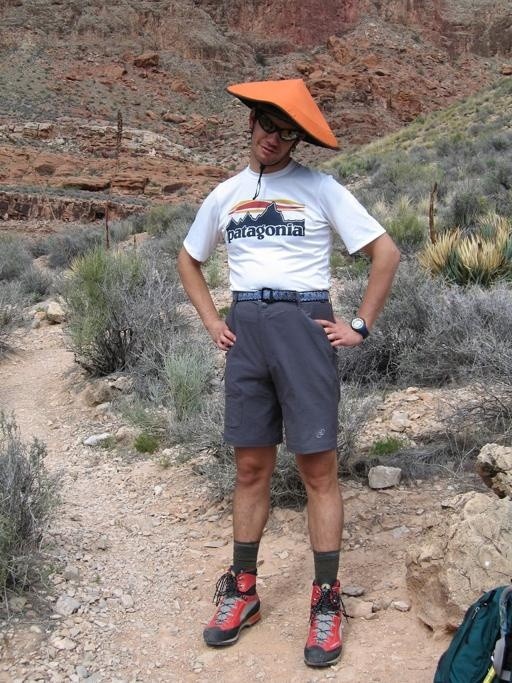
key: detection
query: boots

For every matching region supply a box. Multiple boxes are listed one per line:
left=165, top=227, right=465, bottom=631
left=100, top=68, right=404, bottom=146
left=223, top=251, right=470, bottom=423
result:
left=205, top=569, right=262, bottom=646
left=303, top=583, right=344, bottom=668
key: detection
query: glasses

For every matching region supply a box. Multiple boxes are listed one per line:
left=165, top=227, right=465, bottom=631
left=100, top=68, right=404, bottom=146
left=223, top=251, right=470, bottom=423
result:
left=255, top=112, right=301, bottom=143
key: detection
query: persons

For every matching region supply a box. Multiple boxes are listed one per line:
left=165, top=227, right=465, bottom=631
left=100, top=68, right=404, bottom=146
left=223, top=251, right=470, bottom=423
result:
left=176, top=76, right=401, bottom=670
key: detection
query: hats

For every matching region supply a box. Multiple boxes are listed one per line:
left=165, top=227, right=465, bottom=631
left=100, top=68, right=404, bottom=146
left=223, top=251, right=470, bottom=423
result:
left=227, top=79, right=339, bottom=151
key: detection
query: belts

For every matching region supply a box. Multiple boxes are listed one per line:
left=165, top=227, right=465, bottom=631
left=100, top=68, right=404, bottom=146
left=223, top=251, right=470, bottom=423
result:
left=230, top=290, right=333, bottom=300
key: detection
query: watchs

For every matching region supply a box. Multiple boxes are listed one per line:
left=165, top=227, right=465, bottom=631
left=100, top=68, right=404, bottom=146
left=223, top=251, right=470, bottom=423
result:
left=349, top=318, right=370, bottom=341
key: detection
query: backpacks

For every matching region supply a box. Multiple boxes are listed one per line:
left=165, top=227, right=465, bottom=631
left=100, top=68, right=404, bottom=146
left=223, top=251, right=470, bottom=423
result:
left=434, top=582, right=512, bottom=683
